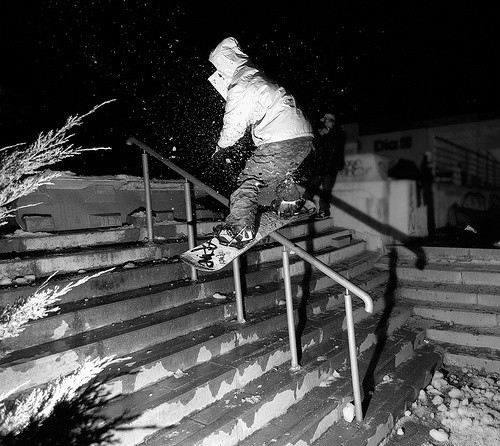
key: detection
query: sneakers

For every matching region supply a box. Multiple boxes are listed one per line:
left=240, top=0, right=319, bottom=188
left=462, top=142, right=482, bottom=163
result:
left=315, top=210, right=330, bottom=218
left=272, top=200, right=299, bottom=216
left=214, top=224, right=253, bottom=242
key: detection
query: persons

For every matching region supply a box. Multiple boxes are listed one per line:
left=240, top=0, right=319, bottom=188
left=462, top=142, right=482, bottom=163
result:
left=207, top=36, right=317, bottom=244
left=302, top=113, right=347, bottom=221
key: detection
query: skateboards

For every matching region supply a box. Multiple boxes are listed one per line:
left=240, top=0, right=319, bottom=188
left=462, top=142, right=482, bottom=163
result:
left=179, top=197, right=318, bottom=273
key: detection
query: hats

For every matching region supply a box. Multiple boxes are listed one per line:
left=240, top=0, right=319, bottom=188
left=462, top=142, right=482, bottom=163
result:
left=320, top=113, right=335, bottom=120
